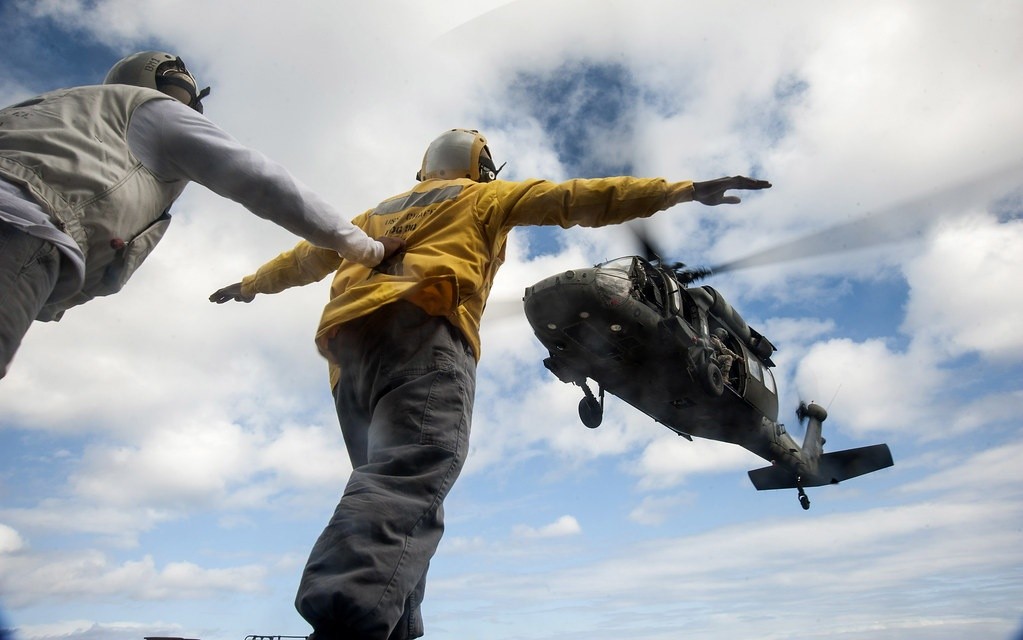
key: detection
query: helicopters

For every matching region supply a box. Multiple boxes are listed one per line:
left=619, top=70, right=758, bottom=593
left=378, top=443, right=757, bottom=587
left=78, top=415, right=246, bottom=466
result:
left=520, top=163, right=1021, bottom=511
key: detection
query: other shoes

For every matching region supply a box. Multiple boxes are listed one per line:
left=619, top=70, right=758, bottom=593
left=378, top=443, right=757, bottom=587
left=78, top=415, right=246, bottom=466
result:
left=723, top=379, right=732, bottom=385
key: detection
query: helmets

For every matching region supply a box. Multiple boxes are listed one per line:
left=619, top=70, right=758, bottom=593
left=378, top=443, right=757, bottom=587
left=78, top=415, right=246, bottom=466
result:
left=103, top=50, right=210, bottom=115
left=716, top=328, right=728, bottom=341
left=420, top=128, right=496, bottom=182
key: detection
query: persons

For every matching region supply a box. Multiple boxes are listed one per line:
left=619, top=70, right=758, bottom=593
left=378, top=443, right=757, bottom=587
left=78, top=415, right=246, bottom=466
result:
left=209, top=130, right=774, bottom=640
left=0, top=51, right=404, bottom=381
